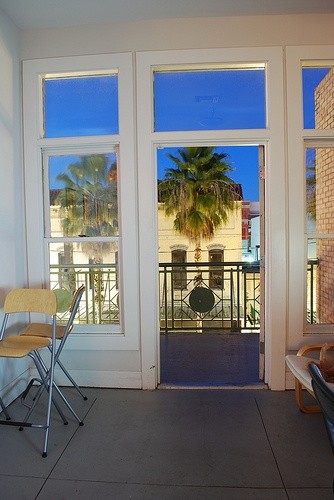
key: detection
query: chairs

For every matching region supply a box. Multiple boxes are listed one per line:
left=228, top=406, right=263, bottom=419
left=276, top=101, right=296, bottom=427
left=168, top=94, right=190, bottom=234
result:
left=0, top=290, right=68, bottom=457
left=19, top=284, right=89, bottom=425
left=308, top=362, right=334, bottom=456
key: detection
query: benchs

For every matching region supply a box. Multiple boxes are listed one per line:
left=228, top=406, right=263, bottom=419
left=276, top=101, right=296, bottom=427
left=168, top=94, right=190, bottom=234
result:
left=285, top=342, right=334, bottom=412
